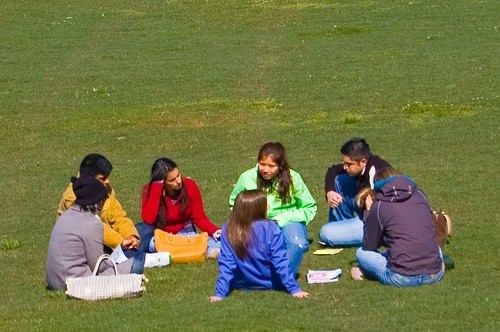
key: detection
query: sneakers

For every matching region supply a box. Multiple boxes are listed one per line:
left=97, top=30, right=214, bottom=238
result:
left=144, top=252, right=172, bottom=268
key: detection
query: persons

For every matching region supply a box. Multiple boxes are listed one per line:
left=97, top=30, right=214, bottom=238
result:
left=209, top=190, right=310, bottom=302
left=228, top=142, right=317, bottom=227
left=46, top=176, right=144, bottom=291
left=135, top=157, right=222, bottom=259
left=318, top=138, right=392, bottom=246
left=57, top=153, right=171, bottom=267
left=350, top=168, right=445, bottom=287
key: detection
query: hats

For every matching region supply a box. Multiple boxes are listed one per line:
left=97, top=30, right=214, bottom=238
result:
left=72, top=177, right=108, bottom=204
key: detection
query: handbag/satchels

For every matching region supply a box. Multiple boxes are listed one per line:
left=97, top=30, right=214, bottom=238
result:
left=65, top=254, right=145, bottom=301
left=153, top=228, right=209, bottom=264
